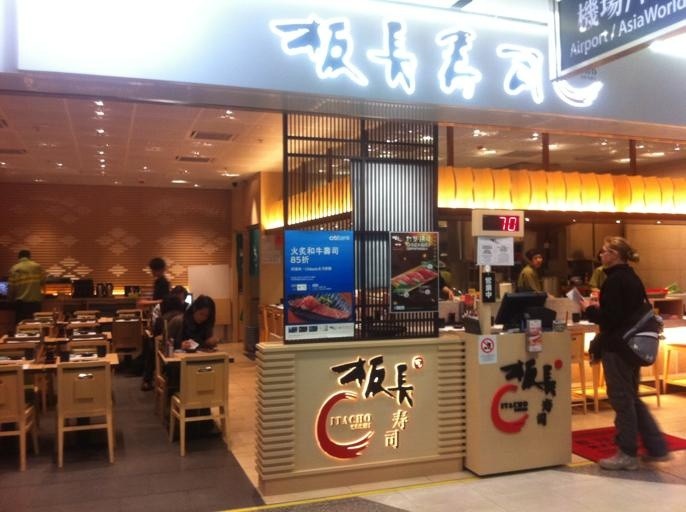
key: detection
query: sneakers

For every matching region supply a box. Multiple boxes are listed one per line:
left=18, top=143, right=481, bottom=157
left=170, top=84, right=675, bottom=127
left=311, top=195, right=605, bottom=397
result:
left=601, top=452, right=639, bottom=471
left=642, top=453, right=671, bottom=462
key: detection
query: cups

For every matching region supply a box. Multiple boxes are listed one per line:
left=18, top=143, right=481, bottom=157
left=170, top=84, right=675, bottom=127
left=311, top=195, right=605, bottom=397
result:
left=97, top=344, right=107, bottom=357
left=7, top=329, right=14, bottom=337
left=25, top=348, right=33, bottom=360
left=60, top=352, right=69, bottom=362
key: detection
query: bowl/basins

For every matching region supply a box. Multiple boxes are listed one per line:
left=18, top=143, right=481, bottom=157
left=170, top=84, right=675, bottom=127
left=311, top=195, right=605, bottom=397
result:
left=81, top=352, right=94, bottom=356
left=24, top=332, right=37, bottom=335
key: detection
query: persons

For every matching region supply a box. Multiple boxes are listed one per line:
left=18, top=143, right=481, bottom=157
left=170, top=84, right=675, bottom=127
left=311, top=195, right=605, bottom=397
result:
left=141, top=285, right=191, bottom=391
left=155, top=299, right=183, bottom=332
left=597, top=236, right=672, bottom=470
left=7, top=249, right=41, bottom=323
left=590, top=263, right=608, bottom=296
left=165, top=295, right=215, bottom=439
left=517, top=249, right=543, bottom=293
left=439, top=275, right=455, bottom=300
left=149, top=258, right=170, bottom=300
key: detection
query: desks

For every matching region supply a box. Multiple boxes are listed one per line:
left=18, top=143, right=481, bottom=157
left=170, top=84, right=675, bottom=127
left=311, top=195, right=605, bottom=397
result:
left=661, top=325, right=686, bottom=399
left=570, top=321, right=595, bottom=416
left=584, top=330, right=664, bottom=414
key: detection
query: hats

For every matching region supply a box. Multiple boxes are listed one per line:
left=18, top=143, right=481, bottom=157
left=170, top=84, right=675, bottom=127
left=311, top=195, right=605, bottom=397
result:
left=524, top=248, right=541, bottom=257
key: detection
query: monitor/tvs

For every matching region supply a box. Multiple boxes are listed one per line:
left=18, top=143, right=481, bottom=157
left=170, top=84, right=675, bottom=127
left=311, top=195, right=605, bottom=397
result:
left=495, top=293, right=548, bottom=330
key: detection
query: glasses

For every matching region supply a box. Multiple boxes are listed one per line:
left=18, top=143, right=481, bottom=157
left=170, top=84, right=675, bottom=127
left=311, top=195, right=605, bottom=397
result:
left=598, top=246, right=610, bottom=255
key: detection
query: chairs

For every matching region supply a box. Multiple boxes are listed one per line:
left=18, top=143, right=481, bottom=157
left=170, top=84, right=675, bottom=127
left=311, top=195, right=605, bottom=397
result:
left=16, top=321, right=55, bottom=340
left=67, top=338, right=111, bottom=356
left=64, top=323, right=102, bottom=339
left=0, top=364, right=40, bottom=473
left=167, top=352, right=229, bottom=457
left=116, top=309, right=144, bottom=336
left=111, top=317, right=144, bottom=364
left=153, top=335, right=169, bottom=422
left=31, top=311, right=56, bottom=322
left=57, top=361, right=115, bottom=470
left=73, top=309, right=101, bottom=320
left=0, top=341, right=39, bottom=361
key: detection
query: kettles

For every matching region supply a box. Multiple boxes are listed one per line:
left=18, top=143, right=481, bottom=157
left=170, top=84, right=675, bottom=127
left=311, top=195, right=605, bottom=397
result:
left=95, top=283, right=113, bottom=296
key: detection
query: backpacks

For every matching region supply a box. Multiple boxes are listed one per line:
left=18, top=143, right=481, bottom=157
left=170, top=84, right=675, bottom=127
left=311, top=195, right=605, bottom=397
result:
left=604, top=267, right=664, bottom=367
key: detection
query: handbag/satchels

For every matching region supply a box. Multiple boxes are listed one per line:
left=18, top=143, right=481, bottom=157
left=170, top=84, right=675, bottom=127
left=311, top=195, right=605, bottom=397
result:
left=613, top=292, right=665, bottom=367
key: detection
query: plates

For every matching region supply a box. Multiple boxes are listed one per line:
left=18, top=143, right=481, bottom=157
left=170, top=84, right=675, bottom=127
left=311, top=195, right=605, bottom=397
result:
left=79, top=330, right=88, bottom=334
left=9, top=356, right=22, bottom=359
left=184, top=349, right=197, bottom=352
left=392, top=264, right=437, bottom=293
